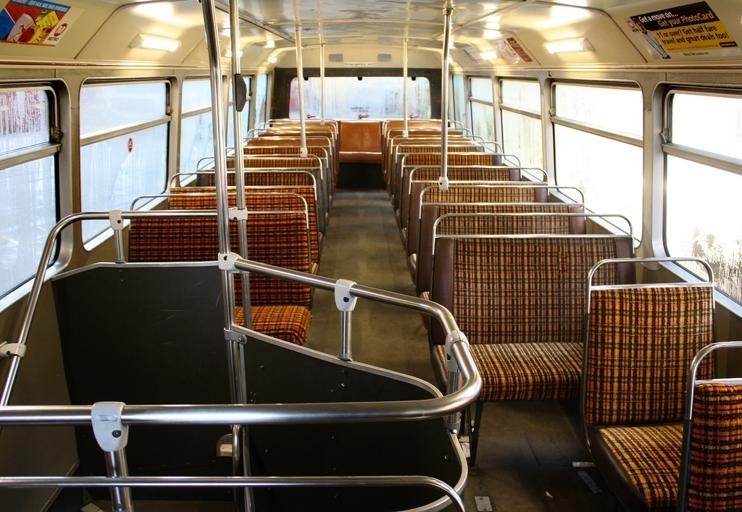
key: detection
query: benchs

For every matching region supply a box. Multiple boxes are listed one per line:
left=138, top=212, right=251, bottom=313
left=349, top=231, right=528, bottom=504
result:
left=128, top=116, right=378, bottom=354
left=377, top=115, right=638, bottom=473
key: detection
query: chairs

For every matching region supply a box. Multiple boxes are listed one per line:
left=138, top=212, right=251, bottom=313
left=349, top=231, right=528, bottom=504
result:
left=569, top=253, right=718, bottom=505
left=674, top=338, right=741, bottom=508
